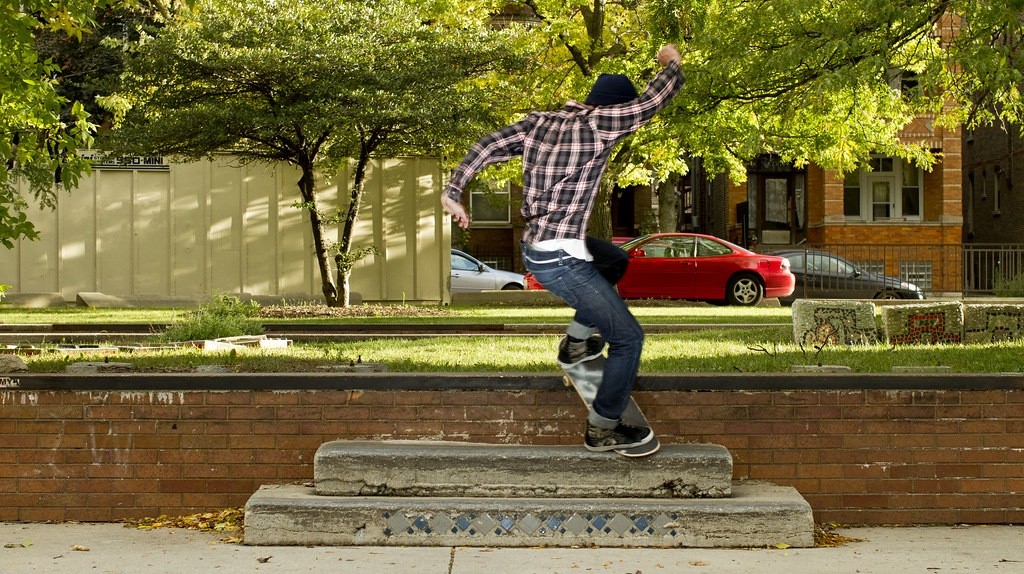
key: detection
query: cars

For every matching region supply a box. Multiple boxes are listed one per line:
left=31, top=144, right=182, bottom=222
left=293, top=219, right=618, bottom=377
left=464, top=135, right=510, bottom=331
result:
left=450, top=247, right=525, bottom=291
left=522, top=237, right=684, bottom=297
left=767, top=250, right=925, bottom=308
left=615, top=232, right=796, bottom=308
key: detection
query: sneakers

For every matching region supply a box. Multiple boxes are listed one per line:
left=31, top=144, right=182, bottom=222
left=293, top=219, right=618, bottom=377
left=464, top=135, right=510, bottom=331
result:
left=582, top=417, right=655, bottom=452
left=556, top=333, right=606, bottom=368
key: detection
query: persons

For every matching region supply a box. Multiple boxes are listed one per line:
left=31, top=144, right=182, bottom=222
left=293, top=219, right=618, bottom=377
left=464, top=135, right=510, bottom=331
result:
left=440, top=43, right=688, bottom=454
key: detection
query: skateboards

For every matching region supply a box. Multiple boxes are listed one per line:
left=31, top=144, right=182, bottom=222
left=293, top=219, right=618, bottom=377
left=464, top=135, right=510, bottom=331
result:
left=557, top=336, right=661, bottom=457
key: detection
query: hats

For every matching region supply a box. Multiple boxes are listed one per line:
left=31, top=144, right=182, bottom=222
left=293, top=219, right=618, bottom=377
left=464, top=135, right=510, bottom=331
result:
left=582, top=73, right=640, bottom=107
